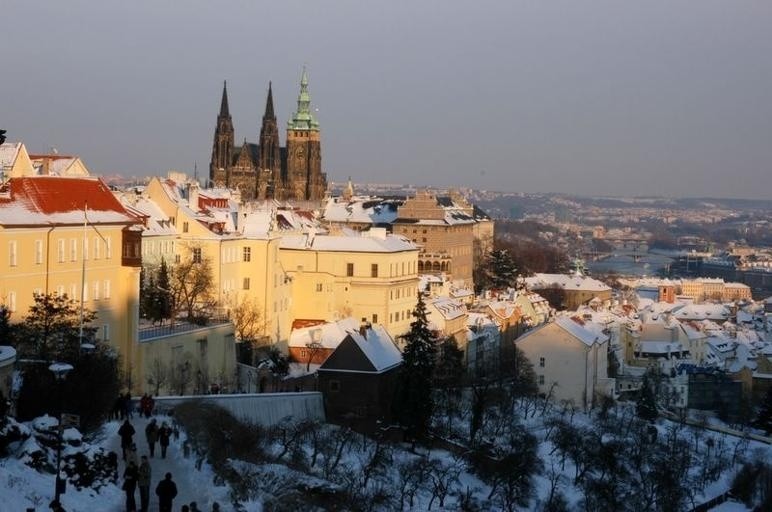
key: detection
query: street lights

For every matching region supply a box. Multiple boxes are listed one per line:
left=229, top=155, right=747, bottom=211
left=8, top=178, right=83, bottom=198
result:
left=49, top=362, right=79, bottom=509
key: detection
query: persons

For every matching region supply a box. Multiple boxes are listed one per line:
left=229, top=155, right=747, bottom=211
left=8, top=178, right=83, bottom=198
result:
left=148, top=393, right=155, bottom=418
left=156, top=472, right=178, bottom=512
left=107, top=391, right=135, bottom=422
left=138, top=456, right=151, bottom=512
left=122, top=461, right=137, bottom=512
left=181, top=504, right=189, bottom=512
left=118, top=419, right=136, bottom=460
left=125, top=442, right=138, bottom=485
left=145, top=419, right=159, bottom=458
left=211, top=502, right=221, bottom=512
left=139, top=392, right=150, bottom=418
left=190, top=501, right=202, bottom=512
left=159, top=421, right=172, bottom=459
